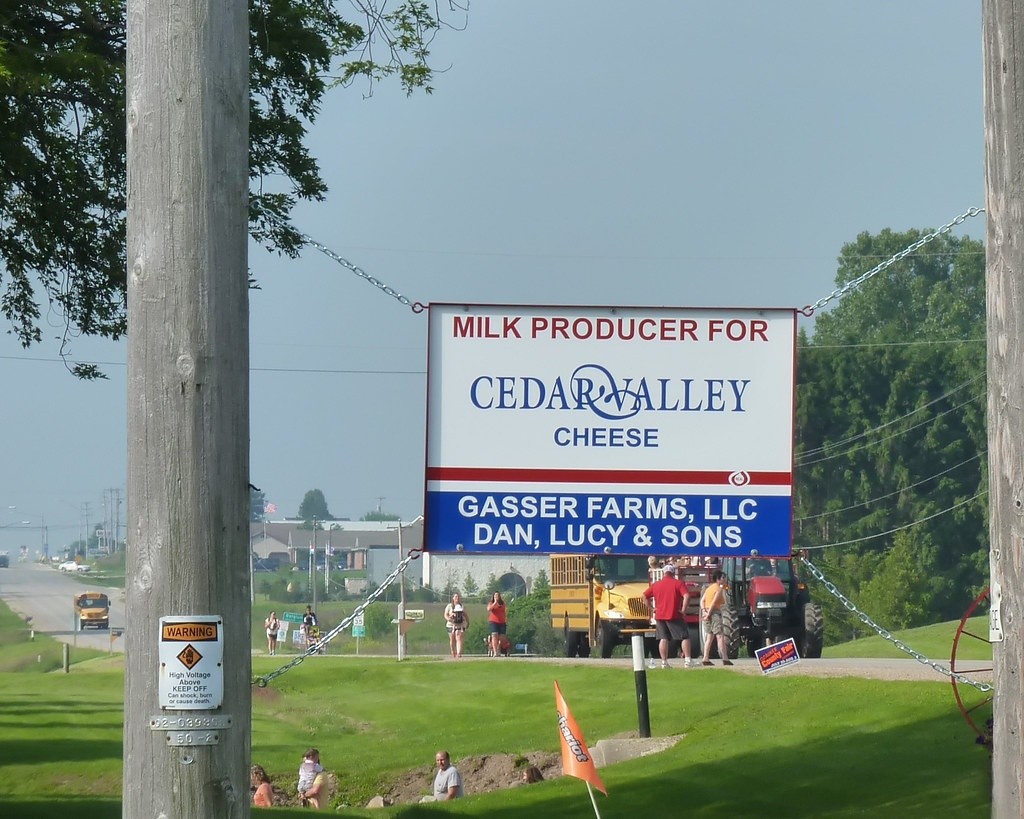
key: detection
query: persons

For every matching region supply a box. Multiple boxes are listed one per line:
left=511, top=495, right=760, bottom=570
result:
left=647, top=555, right=718, bottom=568
left=444, top=592, right=470, bottom=658
left=487, top=591, right=507, bottom=657
left=303, top=606, right=318, bottom=631
left=264, top=611, right=280, bottom=655
left=700, top=571, right=734, bottom=666
left=642, top=565, right=696, bottom=668
left=297, top=749, right=329, bottom=809
left=434, top=751, right=464, bottom=801
left=307, top=629, right=318, bottom=646
left=523, top=767, right=543, bottom=784
left=745, top=558, right=777, bottom=578
left=251, top=764, right=273, bottom=807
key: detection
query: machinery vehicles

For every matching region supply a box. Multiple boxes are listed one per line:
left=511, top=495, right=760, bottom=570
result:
left=647, top=549, right=824, bottom=660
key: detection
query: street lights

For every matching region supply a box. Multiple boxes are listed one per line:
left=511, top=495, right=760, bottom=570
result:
left=0, top=505, right=16, bottom=510
left=0, top=519, right=31, bottom=528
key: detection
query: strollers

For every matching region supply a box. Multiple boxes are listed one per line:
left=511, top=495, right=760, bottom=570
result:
left=300, top=625, right=323, bottom=655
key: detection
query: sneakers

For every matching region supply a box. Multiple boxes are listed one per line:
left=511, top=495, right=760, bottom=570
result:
left=661, top=660, right=671, bottom=671
left=685, top=660, right=700, bottom=667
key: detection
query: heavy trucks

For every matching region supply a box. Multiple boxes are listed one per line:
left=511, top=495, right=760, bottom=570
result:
left=0, top=550, right=9, bottom=569
left=549, top=554, right=656, bottom=658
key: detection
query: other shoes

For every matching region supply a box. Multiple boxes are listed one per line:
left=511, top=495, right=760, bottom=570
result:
left=703, top=661, right=715, bottom=666
left=270, top=650, right=276, bottom=656
left=493, top=650, right=501, bottom=658
left=723, top=659, right=733, bottom=665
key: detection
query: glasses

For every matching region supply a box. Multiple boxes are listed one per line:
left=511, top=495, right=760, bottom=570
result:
left=720, top=577, right=724, bottom=579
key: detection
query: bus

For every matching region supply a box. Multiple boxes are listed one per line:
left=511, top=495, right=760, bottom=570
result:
left=72, top=590, right=112, bottom=630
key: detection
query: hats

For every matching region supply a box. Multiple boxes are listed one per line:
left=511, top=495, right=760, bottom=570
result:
left=662, top=565, right=675, bottom=574
left=648, top=556, right=656, bottom=563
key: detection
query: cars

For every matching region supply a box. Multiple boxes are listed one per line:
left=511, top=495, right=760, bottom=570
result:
left=58, top=561, right=90, bottom=572
